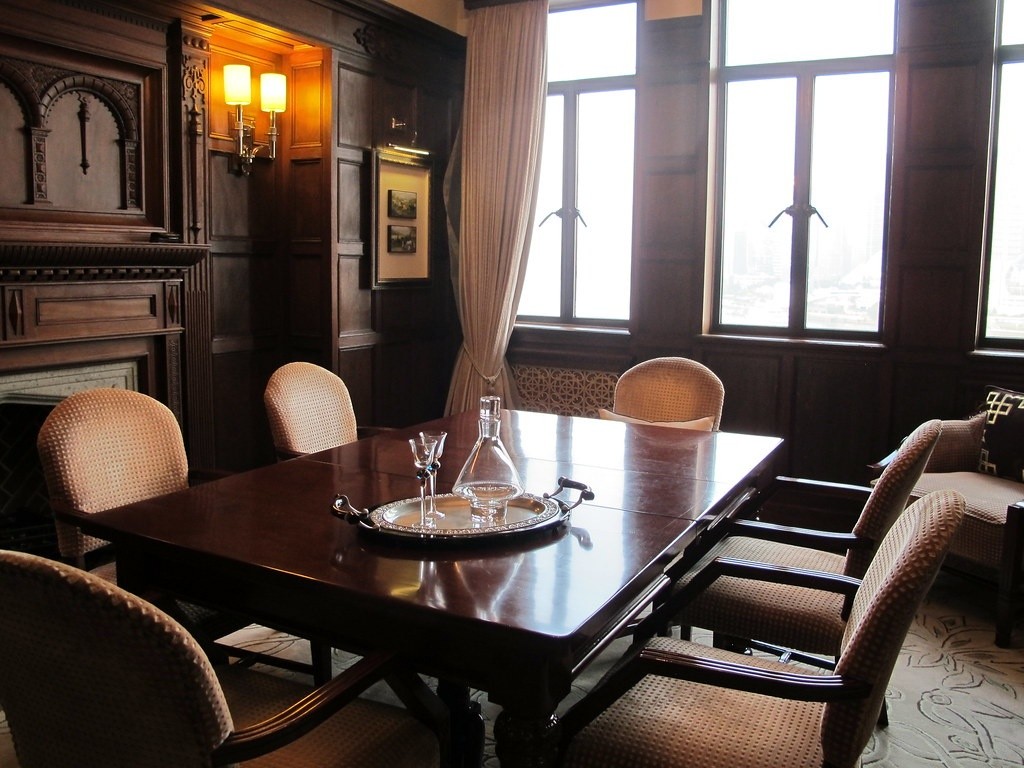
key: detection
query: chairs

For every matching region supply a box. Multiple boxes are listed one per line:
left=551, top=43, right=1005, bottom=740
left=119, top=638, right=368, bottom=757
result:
left=0, top=549, right=452, bottom=768
left=676, top=419, right=945, bottom=728
left=263, top=361, right=400, bottom=465
left=559, top=489, right=965, bottom=768
left=867, top=384, right=1024, bottom=649
left=613, top=357, right=725, bottom=637
left=36, top=388, right=331, bottom=687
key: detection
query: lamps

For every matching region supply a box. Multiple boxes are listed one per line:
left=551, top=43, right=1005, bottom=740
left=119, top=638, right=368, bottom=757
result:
left=223, top=64, right=286, bottom=177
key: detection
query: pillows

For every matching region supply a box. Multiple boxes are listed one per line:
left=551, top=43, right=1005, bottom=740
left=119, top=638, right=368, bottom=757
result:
left=599, top=408, right=715, bottom=432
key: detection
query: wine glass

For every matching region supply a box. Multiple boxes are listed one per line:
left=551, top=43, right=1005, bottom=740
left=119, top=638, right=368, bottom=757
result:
left=419, top=430, right=447, bottom=520
left=409, top=438, right=438, bottom=529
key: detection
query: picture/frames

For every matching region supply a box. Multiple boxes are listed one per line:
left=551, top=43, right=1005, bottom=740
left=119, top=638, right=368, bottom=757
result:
left=371, top=146, right=438, bottom=290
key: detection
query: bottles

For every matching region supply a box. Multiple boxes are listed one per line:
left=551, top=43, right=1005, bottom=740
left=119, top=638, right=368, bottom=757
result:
left=452, top=396, right=526, bottom=524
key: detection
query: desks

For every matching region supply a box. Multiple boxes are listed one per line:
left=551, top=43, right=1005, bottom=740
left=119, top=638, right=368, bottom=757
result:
left=82, top=408, right=789, bottom=768
